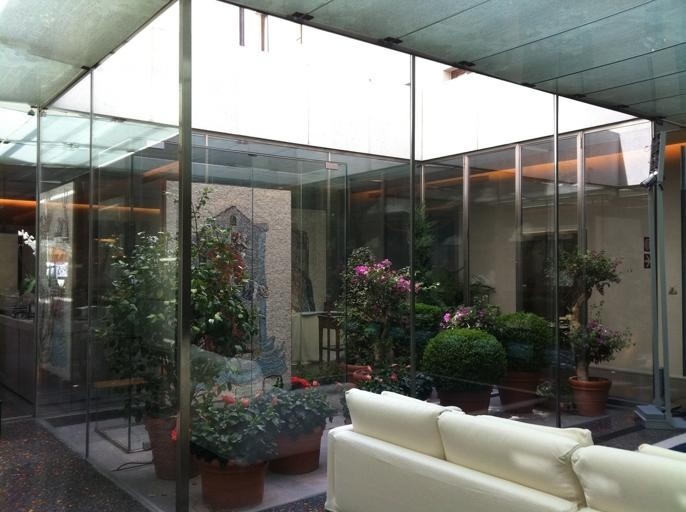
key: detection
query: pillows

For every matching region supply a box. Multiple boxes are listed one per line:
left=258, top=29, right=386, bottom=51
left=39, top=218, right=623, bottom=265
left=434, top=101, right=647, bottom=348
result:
left=474, top=415, right=594, bottom=447
left=436, top=411, right=594, bottom=503
left=638, top=442, right=686, bottom=456
left=344, top=389, right=443, bottom=460
left=569, top=445, right=686, bottom=511
left=381, top=390, right=444, bottom=410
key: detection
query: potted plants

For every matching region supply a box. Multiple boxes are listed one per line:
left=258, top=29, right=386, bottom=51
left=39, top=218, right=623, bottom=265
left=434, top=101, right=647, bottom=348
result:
left=423, top=328, right=507, bottom=414
left=92, top=185, right=258, bottom=481
left=493, top=313, right=550, bottom=414
left=545, top=250, right=632, bottom=417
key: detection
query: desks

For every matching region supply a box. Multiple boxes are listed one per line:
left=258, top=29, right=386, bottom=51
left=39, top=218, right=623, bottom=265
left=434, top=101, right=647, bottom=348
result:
left=317, top=314, right=345, bottom=362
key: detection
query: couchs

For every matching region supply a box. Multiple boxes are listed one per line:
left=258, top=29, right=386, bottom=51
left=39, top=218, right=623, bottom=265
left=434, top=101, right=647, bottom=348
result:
left=321, top=389, right=686, bottom=512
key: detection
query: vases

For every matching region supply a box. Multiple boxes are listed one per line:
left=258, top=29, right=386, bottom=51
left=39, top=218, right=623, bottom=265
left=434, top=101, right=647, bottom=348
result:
left=274, top=430, right=323, bottom=475
left=201, top=462, right=265, bottom=511
left=353, top=369, right=397, bottom=389
left=342, top=364, right=373, bottom=383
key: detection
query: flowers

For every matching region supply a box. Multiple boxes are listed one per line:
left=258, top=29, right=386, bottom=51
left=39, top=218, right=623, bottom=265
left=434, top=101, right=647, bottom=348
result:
left=353, top=364, right=433, bottom=401
left=439, top=306, right=498, bottom=334
left=267, top=376, right=339, bottom=438
left=188, top=393, right=282, bottom=465
left=333, top=247, right=441, bottom=365
